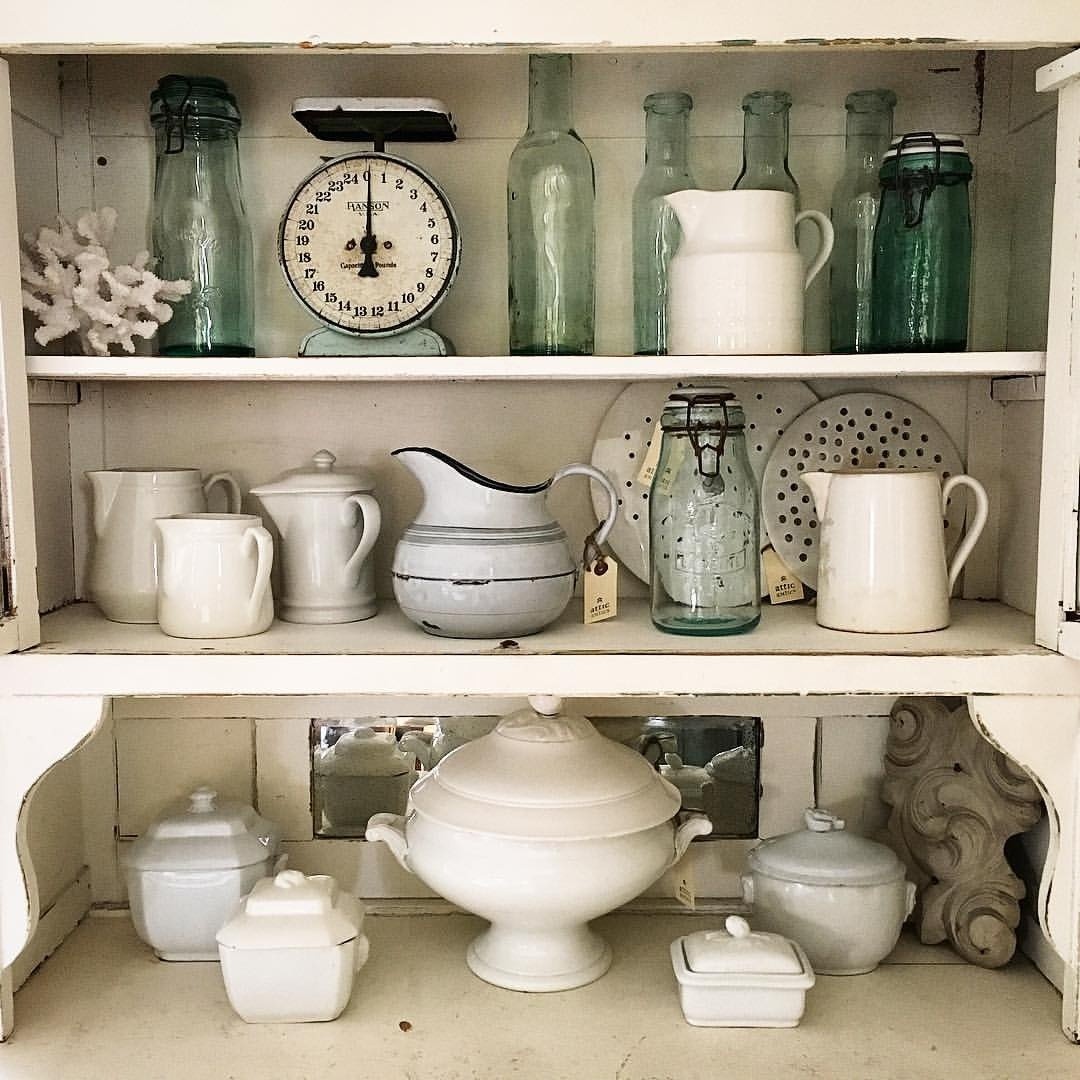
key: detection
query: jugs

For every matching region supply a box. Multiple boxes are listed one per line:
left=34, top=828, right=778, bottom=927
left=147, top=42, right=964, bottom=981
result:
left=661, top=189, right=835, bottom=356
left=800, top=466, right=989, bottom=636
left=249, top=449, right=384, bottom=627
left=390, top=445, right=618, bottom=638
left=150, top=509, right=277, bottom=640
left=84, top=463, right=242, bottom=625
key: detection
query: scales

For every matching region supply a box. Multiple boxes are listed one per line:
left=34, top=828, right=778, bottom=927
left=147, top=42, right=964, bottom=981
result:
left=276, top=96, right=462, bottom=356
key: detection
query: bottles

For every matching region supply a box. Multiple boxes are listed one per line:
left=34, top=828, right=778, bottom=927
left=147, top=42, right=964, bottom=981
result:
left=630, top=91, right=699, bottom=356
left=314, top=728, right=416, bottom=832
left=868, top=131, right=974, bottom=351
left=648, top=385, right=763, bottom=638
left=119, top=785, right=281, bottom=965
left=830, top=89, right=897, bottom=355
left=506, top=54, right=596, bottom=358
left=731, top=90, right=801, bottom=248
left=215, top=868, right=370, bottom=1024
left=146, top=73, right=257, bottom=358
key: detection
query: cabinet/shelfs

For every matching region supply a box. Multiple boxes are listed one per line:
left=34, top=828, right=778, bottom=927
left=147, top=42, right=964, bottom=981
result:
left=0, top=1, right=1080, bottom=1080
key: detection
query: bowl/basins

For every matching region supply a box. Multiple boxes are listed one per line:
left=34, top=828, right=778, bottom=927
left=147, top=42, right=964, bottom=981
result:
left=362, top=696, right=715, bottom=996
left=738, top=807, right=917, bottom=978
left=671, top=915, right=817, bottom=1030
